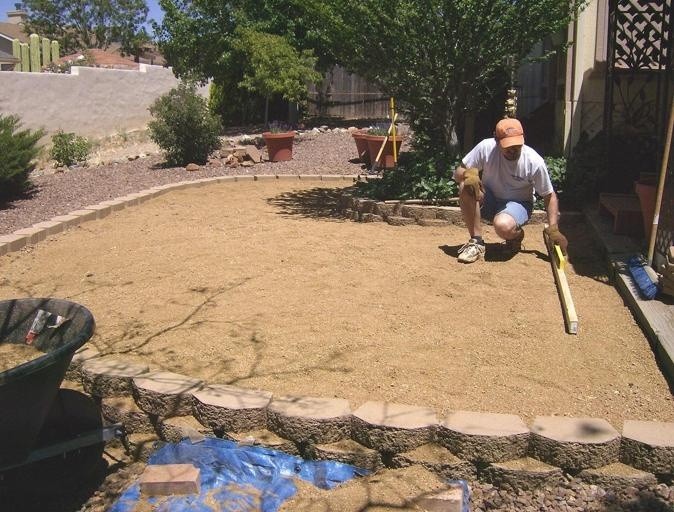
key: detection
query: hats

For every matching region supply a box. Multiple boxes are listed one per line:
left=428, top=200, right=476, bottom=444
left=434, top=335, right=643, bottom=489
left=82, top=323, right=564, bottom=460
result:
left=496, top=119, right=524, bottom=148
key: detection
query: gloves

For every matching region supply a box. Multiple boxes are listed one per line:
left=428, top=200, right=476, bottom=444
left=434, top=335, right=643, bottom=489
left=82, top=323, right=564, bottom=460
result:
left=543, top=227, right=568, bottom=256
left=463, top=168, right=485, bottom=201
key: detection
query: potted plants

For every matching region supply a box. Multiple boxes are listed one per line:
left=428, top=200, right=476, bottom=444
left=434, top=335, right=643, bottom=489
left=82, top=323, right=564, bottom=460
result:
left=352, top=120, right=402, bottom=171
left=262, top=126, right=296, bottom=162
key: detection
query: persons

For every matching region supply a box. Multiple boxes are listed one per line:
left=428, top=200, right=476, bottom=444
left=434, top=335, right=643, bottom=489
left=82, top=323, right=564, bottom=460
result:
left=453, top=116, right=569, bottom=265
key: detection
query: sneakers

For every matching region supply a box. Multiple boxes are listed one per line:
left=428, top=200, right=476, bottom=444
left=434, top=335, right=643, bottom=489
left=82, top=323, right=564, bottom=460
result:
left=458, top=239, right=485, bottom=264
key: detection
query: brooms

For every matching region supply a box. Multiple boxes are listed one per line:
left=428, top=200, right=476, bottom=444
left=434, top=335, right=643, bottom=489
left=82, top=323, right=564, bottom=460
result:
left=364, top=110, right=398, bottom=175
left=626, top=101, right=674, bottom=300
